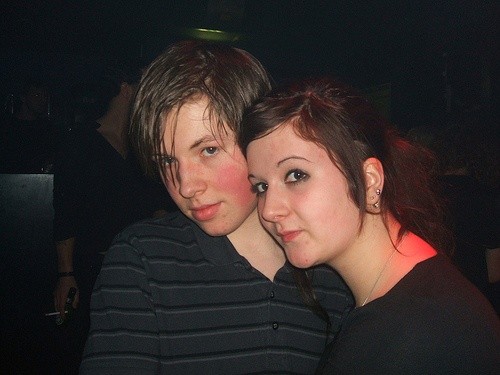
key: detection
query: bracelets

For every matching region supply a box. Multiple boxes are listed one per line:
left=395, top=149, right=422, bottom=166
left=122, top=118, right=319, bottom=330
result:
left=56, top=270, right=75, bottom=278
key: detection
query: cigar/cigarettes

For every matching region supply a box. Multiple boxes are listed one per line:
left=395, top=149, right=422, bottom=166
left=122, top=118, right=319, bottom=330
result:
left=45, top=310, right=68, bottom=316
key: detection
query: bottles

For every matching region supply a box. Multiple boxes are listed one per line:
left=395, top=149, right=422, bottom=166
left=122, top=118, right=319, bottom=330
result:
left=54, top=287, right=77, bottom=330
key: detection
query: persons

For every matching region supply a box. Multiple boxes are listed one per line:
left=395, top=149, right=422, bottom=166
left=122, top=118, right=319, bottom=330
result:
left=49, top=68, right=184, bottom=343
left=0, top=74, right=98, bottom=174
left=244, top=77, right=500, bottom=375
left=430, top=149, right=474, bottom=212
left=479, top=192, right=500, bottom=284
left=81, top=33, right=357, bottom=375
